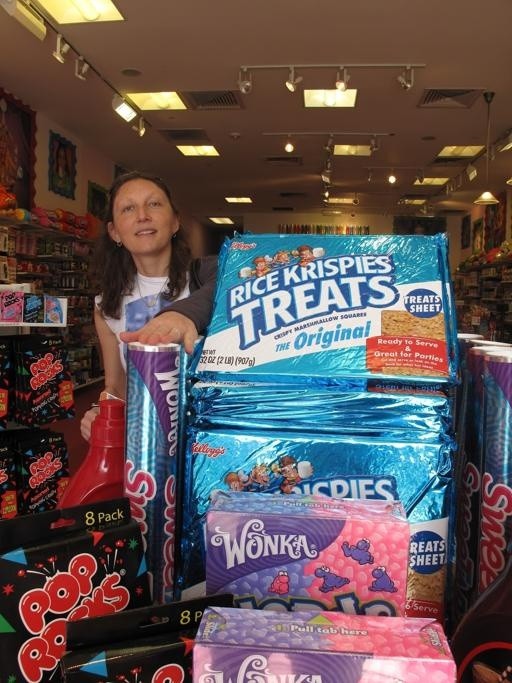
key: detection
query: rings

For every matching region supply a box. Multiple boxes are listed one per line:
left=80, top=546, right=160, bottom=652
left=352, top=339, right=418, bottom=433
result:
left=172, top=328, right=182, bottom=344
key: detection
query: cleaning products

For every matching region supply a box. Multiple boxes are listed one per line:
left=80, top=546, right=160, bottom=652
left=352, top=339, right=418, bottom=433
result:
left=50, top=399, right=127, bottom=526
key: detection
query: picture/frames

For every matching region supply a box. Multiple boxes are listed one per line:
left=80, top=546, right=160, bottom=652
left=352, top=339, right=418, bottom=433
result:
left=0, top=86, right=39, bottom=212
left=86, top=180, right=106, bottom=220
left=47, top=127, right=78, bottom=201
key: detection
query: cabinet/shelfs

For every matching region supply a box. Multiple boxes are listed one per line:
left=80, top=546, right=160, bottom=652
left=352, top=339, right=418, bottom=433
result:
left=455, top=255, right=512, bottom=339
left=0, top=222, right=108, bottom=392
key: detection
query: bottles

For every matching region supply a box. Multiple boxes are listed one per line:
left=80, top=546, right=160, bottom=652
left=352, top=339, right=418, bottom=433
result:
left=54, top=398, right=125, bottom=508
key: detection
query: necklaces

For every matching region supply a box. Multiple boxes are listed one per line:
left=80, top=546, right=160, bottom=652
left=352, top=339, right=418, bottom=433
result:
left=136, top=274, right=169, bottom=309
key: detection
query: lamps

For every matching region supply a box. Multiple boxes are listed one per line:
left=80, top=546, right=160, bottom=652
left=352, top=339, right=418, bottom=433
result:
left=233, top=63, right=417, bottom=93
left=51, top=32, right=91, bottom=82
left=473, top=90, right=499, bottom=205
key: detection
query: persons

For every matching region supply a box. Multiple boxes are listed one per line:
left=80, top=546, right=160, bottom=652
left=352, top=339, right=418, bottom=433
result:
left=118, top=276, right=219, bottom=356
left=78, top=167, right=192, bottom=445
